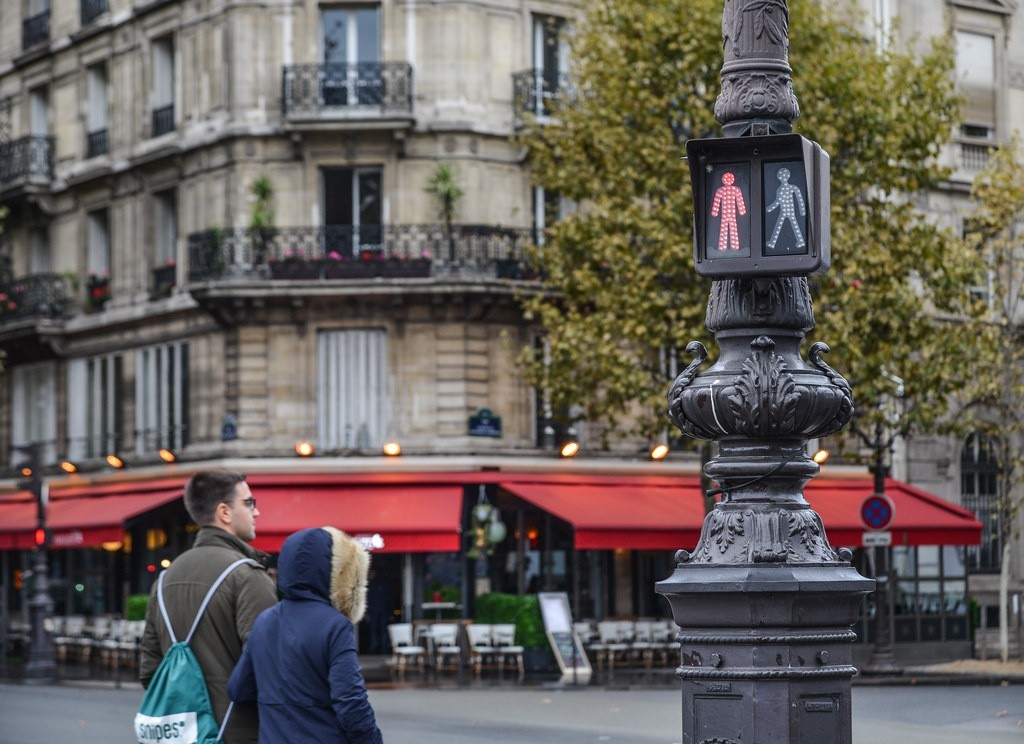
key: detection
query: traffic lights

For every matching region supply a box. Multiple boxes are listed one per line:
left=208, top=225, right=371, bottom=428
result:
left=34, top=525, right=54, bottom=547
left=685, top=133, right=833, bottom=275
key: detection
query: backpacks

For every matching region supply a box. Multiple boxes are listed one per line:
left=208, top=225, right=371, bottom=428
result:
left=133, top=555, right=268, bottom=744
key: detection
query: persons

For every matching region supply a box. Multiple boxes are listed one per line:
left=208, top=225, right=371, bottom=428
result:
left=225, top=525, right=384, bottom=744
left=137, top=469, right=278, bottom=743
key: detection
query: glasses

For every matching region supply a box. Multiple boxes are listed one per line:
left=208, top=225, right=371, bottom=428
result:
left=225, top=498, right=257, bottom=510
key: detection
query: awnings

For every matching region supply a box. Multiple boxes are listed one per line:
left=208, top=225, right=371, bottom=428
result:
left=495, top=473, right=984, bottom=551
left=244, top=474, right=465, bottom=553
left=0, top=477, right=193, bottom=549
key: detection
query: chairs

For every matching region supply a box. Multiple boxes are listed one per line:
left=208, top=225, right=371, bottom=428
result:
left=386, top=620, right=525, bottom=681
left=573, top=617, right=681, bottom=669
left=46, top=613, right=151, bottom=691
